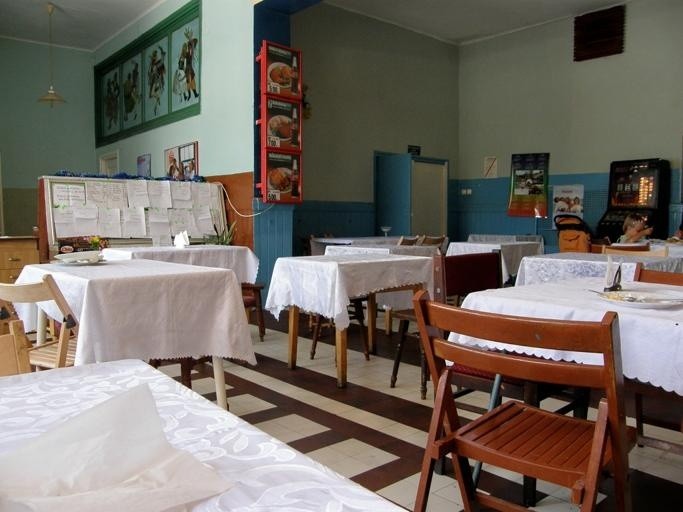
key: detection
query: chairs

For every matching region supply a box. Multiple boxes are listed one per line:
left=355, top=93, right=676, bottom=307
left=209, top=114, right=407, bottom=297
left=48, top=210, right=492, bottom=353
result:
left=1, top=274, right=80, bottom=370
left=411, top=288, right=637, bottom=511
left=1, top=320, right=36, bottom=377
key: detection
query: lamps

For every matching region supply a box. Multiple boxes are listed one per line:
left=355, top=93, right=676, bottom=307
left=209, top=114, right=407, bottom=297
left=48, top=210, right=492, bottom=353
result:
left=37, top=3, right=67, bottom=109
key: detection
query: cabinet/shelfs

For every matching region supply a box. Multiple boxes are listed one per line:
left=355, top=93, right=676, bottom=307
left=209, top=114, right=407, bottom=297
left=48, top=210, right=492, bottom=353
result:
left=1, top=236, right=39, bottom=284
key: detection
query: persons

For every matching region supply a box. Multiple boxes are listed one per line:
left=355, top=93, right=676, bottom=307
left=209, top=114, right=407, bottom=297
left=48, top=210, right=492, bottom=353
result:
left=172, top=27, right=199, bottom=103
left=148, top=42, right=166, bottom=116
left=617, top=213, right=653, bottom=244
left=124, top=62, right=140, bottom=122
left=554, top=196, right=583, bottom=213
left=105, top=72, right=120, bottom=130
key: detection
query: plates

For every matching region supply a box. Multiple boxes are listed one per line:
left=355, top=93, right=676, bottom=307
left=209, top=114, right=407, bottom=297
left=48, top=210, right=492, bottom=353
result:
left=611, top=242, right=646, bottom=248
left=268, top=62, right=292, bottom=88
left=598, top=291, right=683, bottom=309
left=267, top=115, right=292, bottom=141
left=267, top=167, right=292, bottom=194
left=54, top=251, right=101, bottom=262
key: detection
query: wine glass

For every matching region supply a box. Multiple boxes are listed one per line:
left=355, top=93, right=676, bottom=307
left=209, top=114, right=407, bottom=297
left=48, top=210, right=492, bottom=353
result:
left=381, top=226, right=392, bottom=237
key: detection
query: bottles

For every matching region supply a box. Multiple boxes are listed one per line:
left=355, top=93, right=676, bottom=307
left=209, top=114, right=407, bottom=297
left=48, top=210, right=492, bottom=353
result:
left=291, top=105, right=298, bottom=148
left=292, top=159, right=299, bottom=198
left=291, top=56, right=299, bottom=96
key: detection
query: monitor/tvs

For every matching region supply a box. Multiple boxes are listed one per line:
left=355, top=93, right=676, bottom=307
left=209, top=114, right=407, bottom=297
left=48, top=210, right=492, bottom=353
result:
left=608, top=158, right=670, bottom=210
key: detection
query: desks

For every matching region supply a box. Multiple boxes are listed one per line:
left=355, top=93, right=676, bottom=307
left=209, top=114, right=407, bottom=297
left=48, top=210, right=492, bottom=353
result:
left=1, top=359, right=411, bottom=511
left=12, top=233, right=683, bottom=411
